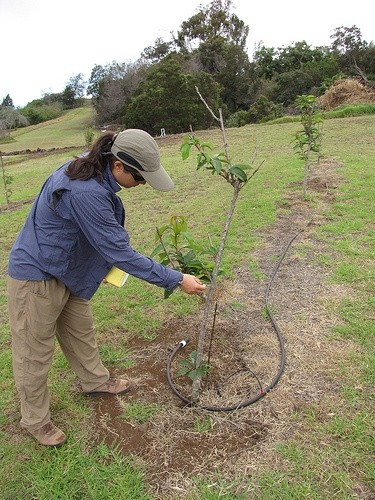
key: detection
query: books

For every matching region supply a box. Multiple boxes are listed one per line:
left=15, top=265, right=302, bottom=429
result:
left=103, top=266, right=130, bottom=287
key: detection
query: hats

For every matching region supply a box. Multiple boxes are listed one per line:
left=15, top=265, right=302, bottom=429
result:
left=112, top=129, right=174, bottom=190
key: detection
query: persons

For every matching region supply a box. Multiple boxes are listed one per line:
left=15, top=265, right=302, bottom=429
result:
left=6, top=128, right=206, bottom=447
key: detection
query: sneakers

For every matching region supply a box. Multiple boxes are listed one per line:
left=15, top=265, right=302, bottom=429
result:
left=84, top=378, right=131, bottom=396
left=25, top=420, right=67, bottom=447
